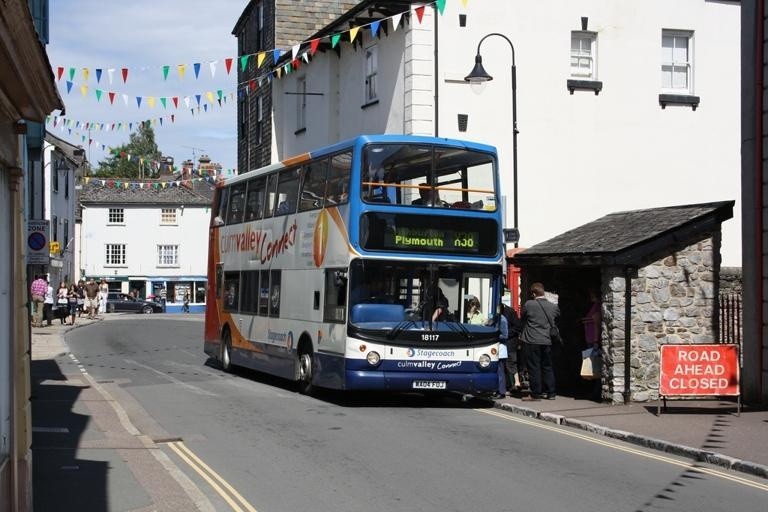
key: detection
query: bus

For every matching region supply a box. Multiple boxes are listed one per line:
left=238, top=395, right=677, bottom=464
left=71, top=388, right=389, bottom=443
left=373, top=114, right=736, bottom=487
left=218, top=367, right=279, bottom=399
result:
left=204, top=134, right=507, bottom=394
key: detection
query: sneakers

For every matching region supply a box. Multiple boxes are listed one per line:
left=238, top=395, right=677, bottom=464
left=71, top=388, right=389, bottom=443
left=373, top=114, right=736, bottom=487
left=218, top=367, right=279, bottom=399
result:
left=522, top=394, right=541, bottom=401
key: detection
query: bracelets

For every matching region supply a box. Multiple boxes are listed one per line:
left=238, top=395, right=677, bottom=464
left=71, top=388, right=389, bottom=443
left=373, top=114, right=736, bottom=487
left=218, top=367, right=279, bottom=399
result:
left=592, top=340, right=599, bottom=344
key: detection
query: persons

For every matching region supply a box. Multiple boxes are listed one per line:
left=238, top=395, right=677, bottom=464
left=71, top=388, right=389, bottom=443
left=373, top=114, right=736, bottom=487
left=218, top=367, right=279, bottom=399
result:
left=431, top=287, right=449, bottom=323
left=129, top=289, right=139, bottom=299
left=468, top=297, right=521, bottom=399
left=585, top=287, right=601, bottom=352
left=426, top=190, right=444, bottom=208
left=159, top=285, right=167, bottom=313
left=31, top=276, right=109, bottom=326
left=518, top=282, right=561, bottom=401
left=183, top=290, right=189, bottom=313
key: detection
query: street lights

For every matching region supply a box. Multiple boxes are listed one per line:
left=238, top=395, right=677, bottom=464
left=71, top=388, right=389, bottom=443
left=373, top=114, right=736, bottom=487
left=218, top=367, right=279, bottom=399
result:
left=27, top=145, right=38, bottom=356
left=464, top=32, right=519, bottom=248
left=41, top=144, right=71, bottom=218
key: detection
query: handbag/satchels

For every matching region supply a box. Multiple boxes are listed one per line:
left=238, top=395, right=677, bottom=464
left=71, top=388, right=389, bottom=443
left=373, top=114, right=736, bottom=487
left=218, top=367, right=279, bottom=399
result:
left=550, top=325, right=562, bottom=345
left=581, top=356, right=602, bottom=379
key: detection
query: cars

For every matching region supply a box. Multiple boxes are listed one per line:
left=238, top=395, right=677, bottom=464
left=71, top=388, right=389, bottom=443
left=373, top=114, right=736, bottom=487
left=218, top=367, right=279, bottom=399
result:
left=106, top=292, right=163, bottom=314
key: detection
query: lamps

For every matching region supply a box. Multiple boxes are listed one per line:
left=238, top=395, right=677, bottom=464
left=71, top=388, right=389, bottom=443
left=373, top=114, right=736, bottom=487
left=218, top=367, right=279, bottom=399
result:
left=41, top=141, right=70, bottom=178
